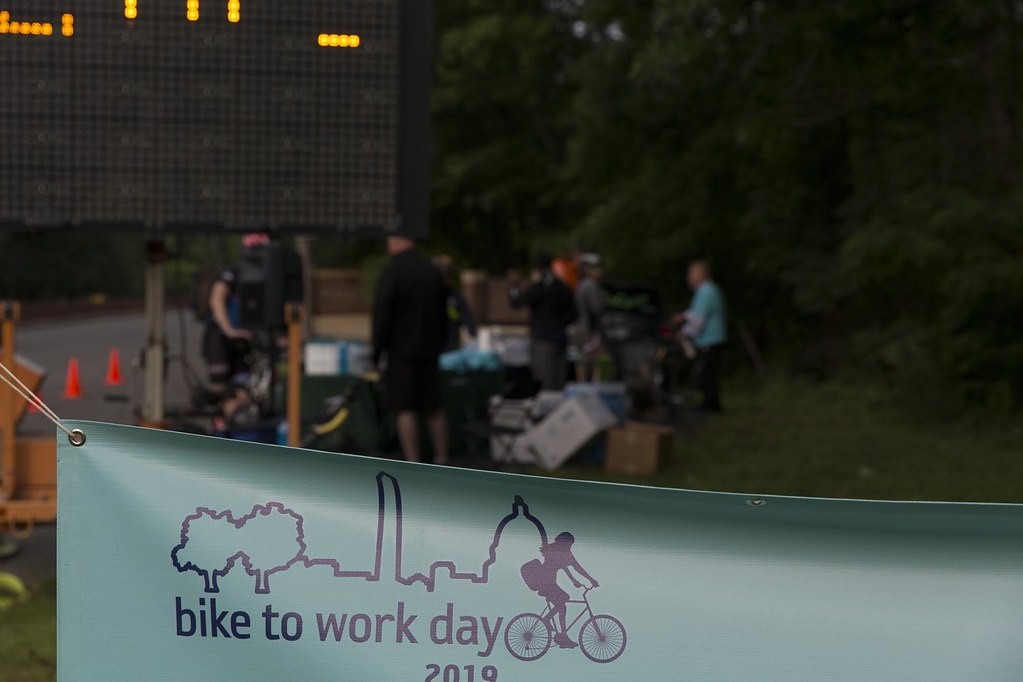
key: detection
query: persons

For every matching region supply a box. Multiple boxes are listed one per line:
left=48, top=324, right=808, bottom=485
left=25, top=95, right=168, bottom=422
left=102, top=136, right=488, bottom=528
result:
left=510, top=249, right=603, bottom=387
left=203, top=243, right=303, bottom=389
left=369, top=230, right=453, bottom=466
left=672, top=258, right=730, bottom=413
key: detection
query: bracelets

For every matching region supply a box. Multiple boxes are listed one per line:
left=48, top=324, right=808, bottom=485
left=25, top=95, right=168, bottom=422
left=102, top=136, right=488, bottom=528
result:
left=680, top=312, right=685, bottom=322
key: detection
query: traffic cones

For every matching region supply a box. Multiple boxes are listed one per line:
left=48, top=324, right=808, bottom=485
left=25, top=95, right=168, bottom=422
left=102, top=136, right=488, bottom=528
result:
left=61, top=358, right=83, bottom=399
left=26, top=390, right=46, bottom=414
left=103, top=348, right=127, bottom=387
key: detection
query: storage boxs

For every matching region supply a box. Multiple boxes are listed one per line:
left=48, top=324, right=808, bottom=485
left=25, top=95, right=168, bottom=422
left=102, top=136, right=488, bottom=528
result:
left=460, top=325, right=532, bottom=366
left=515, top=386, right=617, bottom=472
left=302, top=315, right=375, bottom=377
left=605, top=419, right=674, bottom=475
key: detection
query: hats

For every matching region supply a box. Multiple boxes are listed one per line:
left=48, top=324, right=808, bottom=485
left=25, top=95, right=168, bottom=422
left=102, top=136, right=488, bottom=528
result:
left=383, top=223, right=429, bottom=242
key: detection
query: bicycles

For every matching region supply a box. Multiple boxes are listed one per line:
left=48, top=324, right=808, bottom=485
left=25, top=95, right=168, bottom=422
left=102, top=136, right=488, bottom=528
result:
left=137, top=333, right=307, bottom=445
left=302, top=359, right=402, bottom=460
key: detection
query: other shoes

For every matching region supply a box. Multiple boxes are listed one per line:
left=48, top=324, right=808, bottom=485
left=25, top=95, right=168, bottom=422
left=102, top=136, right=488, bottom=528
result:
left=697, top=402, right=722, bottom=413
left=434, top=450, right=450, bottom=465
